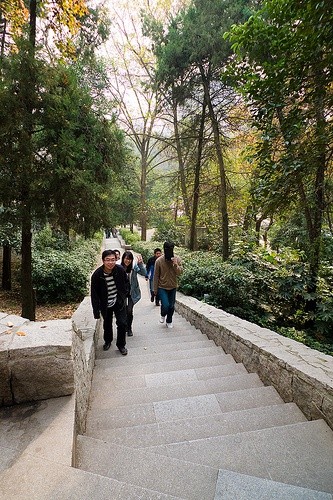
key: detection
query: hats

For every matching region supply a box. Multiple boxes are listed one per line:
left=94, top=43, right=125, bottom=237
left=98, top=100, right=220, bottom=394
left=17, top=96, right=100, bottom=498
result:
left=165, top=248, right=174, bottom=258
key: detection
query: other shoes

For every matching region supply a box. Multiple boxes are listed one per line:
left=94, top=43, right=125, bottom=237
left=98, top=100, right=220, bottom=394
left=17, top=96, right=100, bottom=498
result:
left=128, top=330, right=133, bottom=336
left=160, top=316, right=165, bottom=323
left=167, top=322, right=173, bottom=328
left=118, top=347, right=128, bottom=355
left=151, top=298, right=154, bottom=302
left=103, top=342, right=111, bottom=350
left=156, top=302, right=160, bottom=306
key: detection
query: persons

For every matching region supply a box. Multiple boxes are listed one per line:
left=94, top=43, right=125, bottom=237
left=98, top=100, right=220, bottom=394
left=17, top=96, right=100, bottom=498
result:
left=106, top=228, right=118, bottom=239
left=91, top=250, right=131, bottom=356
left=114, top=250, right=148, bottom=336
left=144, top=247, right=161, bottom=306
left=153, top=241, right=183, bottom=328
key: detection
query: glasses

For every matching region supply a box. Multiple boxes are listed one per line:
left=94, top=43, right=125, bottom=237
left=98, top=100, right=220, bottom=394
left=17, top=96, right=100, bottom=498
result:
left=104, top=260, right=115, bottom=262
